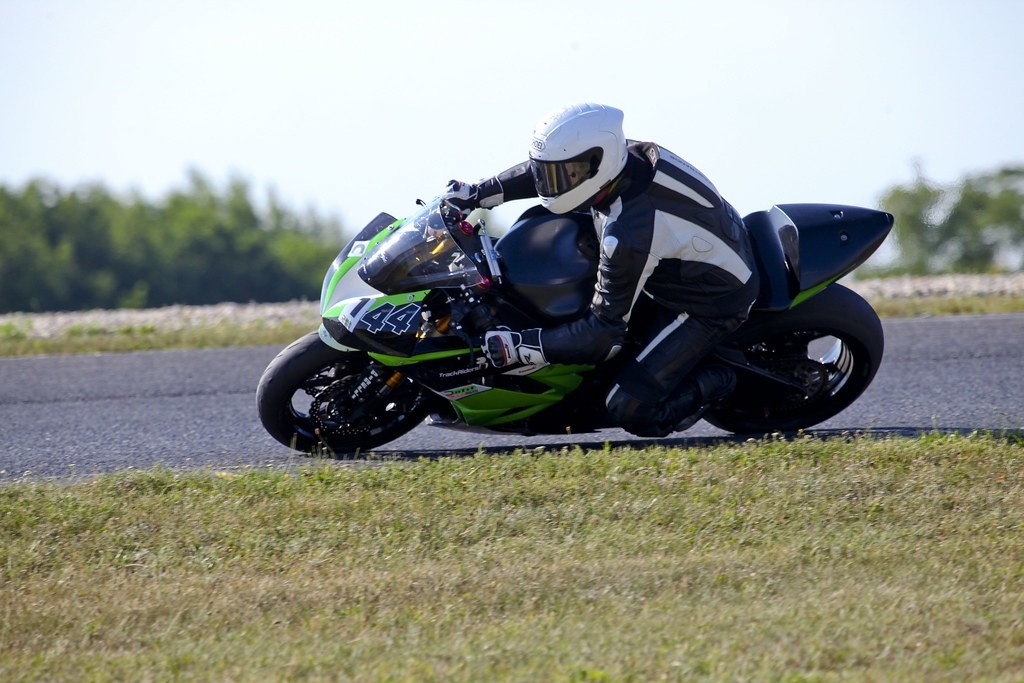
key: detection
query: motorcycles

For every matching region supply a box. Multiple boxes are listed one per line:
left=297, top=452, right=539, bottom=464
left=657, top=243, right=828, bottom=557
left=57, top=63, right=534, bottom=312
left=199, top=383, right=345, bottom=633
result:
left=250, top=177, right=894, bottom=460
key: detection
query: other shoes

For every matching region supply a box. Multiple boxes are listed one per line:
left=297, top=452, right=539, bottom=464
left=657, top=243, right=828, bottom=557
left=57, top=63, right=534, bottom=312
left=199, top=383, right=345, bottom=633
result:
left=673, top=364, right=736, bottom=432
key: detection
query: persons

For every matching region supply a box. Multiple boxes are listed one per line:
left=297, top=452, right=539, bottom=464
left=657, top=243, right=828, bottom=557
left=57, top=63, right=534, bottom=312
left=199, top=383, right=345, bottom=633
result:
left=445, top=100, right=760, bottom=434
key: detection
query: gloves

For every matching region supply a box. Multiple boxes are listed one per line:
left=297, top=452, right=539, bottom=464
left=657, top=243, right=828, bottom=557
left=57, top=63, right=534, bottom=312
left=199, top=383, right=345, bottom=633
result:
left=476, top=324, right=550, bottom=373
left=427, top=179, right=504, bottom=229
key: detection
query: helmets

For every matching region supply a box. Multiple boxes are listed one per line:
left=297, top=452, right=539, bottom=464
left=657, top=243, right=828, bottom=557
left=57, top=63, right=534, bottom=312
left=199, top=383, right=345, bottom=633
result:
left=530, top=101, right=628, bottom=215
